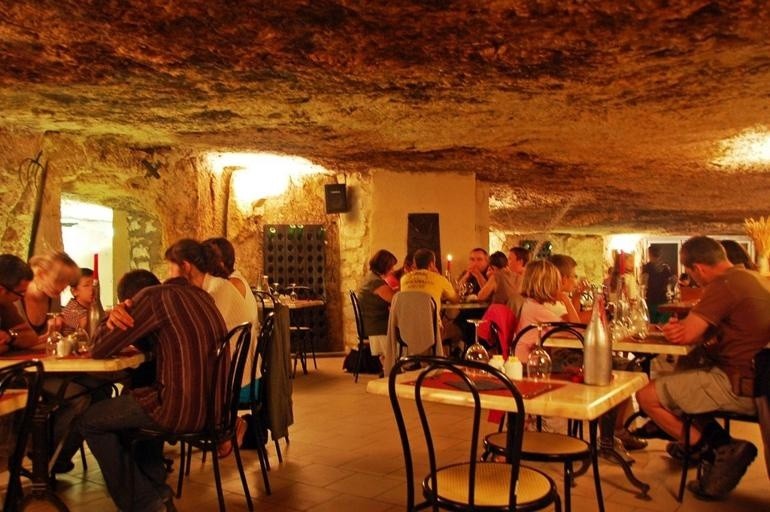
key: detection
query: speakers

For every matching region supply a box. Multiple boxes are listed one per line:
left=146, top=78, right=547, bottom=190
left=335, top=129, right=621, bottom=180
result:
left=325, top=184, right=346, bottom=212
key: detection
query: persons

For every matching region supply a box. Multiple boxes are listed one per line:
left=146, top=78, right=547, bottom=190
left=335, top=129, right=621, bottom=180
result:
left=508, top=233, right=769, bottom=502
left=1, top=232, right=263, bottom=512
left=354, top=246, right=536, bottom=372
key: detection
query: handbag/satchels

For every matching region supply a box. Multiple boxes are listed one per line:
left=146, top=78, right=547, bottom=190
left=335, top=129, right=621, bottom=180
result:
left=341, top=350, right=381, bottom=373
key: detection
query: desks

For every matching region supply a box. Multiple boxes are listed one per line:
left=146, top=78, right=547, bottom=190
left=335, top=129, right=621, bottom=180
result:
left=366, top=361, right=652, bottom=512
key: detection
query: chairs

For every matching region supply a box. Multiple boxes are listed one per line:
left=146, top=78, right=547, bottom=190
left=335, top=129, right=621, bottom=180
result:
left=187, top=312, right=290, bottom=478
left=242, top=278, right=324, bottom=377
left=388, top=352, right=568, bottom=512
left=0, top=358, right=50, bottom=511
left=121, top=322, right=254, bottom=512
left=349, top=274, right=768, bottom=503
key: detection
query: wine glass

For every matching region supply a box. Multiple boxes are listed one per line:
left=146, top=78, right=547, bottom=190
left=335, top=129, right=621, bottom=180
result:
left=41, top=307, right=103, bottom=361
left=604, top=273, right=682, bottom=342
left=526, top=318, right=554, bottom=383
left=446, top=277, right=474, bottom=301
left=463, top=318, right=491, bottom=381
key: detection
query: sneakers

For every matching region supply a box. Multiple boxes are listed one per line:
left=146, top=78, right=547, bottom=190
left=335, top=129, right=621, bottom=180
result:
left=666, top=439, right=757, bottom=499
left=597, top=430, right=648, bottom=465
left=217, top=416, right=249, bottom=458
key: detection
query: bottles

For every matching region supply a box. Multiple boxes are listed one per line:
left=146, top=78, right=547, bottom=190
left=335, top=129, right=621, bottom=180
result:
left=504, top=354, right=523, bottom=383
left=251, top=274, right=298, bottom=308
left=582, top=281, right=615, bottom=388
left=490, top=354, right=506, bottom=380
